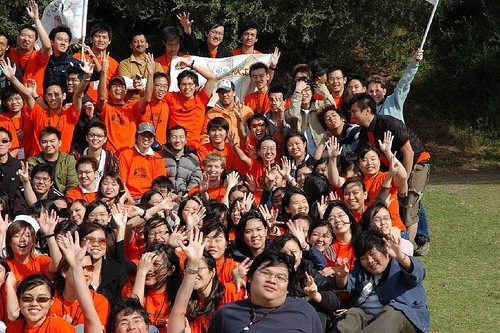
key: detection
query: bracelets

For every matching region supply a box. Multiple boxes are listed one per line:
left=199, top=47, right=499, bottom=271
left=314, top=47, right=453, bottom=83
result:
left=140, top=211, right=147, bottom=218
left=191, top=59, right=194, bottom=70
left=43, top=234, right=56, bottom=238
left=399, top=255, right=407, bottom=264
left=269, top=66, right=277, bottom=70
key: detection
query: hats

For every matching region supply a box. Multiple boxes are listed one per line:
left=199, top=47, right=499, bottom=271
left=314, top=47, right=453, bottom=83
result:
left=215, top=78, right=235, bottom=93
left=136, top=122, right=156, bottom=136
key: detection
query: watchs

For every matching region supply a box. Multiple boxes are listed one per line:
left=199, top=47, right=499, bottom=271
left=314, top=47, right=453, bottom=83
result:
left=185, top=266, right=198, bottom=275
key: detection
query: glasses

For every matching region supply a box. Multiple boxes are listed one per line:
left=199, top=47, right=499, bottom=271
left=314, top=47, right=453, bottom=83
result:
left=255, top=268, right=288, bottom=283
left=88, top=133, right=105, bottom=138
left=19, top=296, right=51, bottom=302
left=250, top=122, right=265, bottom=128
left=77, top=170, right=94, bottom=175
left=1, top=139, right=11, bottom=143
left=147, top=230, right=169, bottom=239
left=182, top=266, right=209, bottom=275
left=81, top=236, right=107, bottom=247
left=82, top=265, right=95, bottom=272
left=153, top=84, right=168, bottom=89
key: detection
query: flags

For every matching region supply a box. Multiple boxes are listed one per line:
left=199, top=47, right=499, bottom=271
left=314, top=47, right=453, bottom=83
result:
left=28, top=0, right=88, bottom=55
left=169, top=52, right=275, bottom=105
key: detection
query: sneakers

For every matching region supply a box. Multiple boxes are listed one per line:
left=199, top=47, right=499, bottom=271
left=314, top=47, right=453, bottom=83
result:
left=414, top=238, right=430, bottom=256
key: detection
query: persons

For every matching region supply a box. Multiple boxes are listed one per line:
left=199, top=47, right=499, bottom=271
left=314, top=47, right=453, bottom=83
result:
left=326, top=229, right=430, bottom=333
left=0, top=0, right=432, bottom=333
left=207, top=250, right=326, bottom=333
left=3, top=274, right=78, bottom=333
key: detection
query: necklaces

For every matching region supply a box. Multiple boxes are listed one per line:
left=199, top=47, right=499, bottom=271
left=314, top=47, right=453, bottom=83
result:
left=241, top=299, right=282, bottom=333
